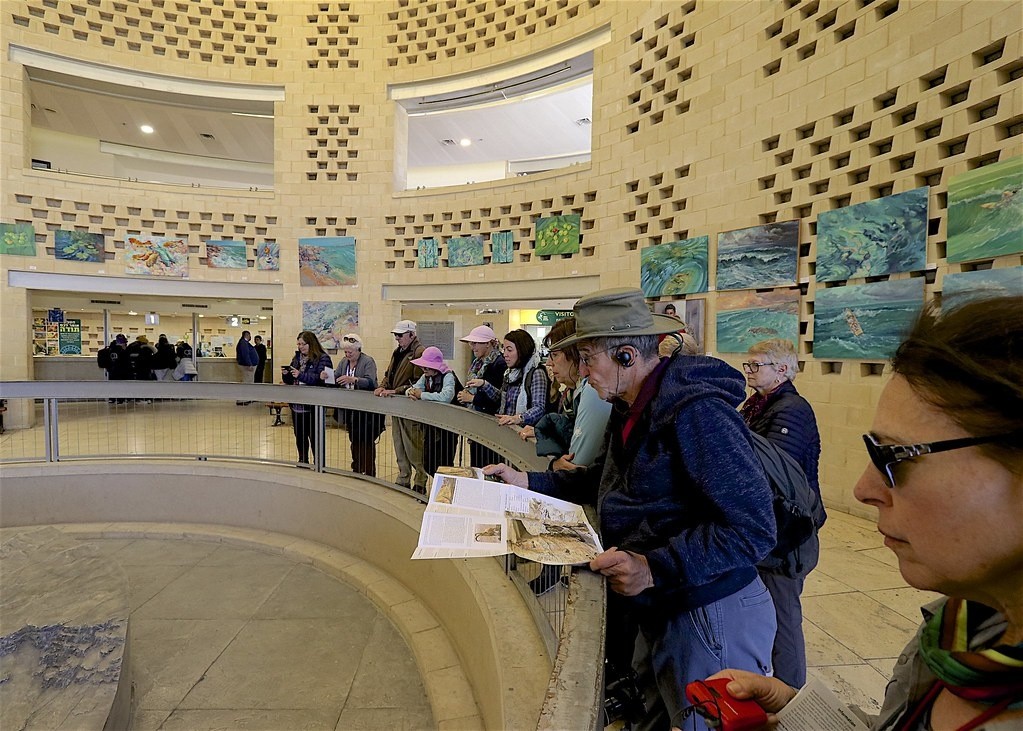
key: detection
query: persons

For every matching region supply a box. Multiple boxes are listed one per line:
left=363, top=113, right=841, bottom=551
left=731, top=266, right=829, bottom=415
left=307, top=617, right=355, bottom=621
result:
left=105, top=333, right=192, bottom=403
left=405, top=350, right=462, bottom=504
left=704, top=287, right=1022, bottom=730
left=236, top=331, right=259, bottom=406
left=254, top=335, right=266, bottom=383
left=319, top=334, right=383, bottom=477
left=281, top=330, right=333, bottom=472
left=374, top=320, right=428, bottom=496
left=735, top=337, right=826, bottom=689
left=478, top=292, right=780, bottom=730
left=457, top=325, right=701, bottom=598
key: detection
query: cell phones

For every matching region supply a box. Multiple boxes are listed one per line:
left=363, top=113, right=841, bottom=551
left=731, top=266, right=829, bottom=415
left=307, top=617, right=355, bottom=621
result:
left=281, top=366, right=292, bottom=371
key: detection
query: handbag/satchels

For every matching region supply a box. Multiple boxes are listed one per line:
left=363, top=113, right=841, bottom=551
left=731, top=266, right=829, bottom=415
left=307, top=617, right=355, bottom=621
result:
left=96, top=348, right=113, bottom=369
left=451, top=370, right=469, bottom=409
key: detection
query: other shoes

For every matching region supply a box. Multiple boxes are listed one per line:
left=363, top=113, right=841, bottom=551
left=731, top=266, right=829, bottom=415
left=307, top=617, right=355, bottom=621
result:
left=562, top=575, right=572, bottom=588
left=394, top=481, right=411, bottom=493
left=526, top=569, right=559, bottom=596
left=236, top=401, right=250, bottom=406
left=108, top=398, right=152, bottom=406
left=411, top=484, right=427, bottom=498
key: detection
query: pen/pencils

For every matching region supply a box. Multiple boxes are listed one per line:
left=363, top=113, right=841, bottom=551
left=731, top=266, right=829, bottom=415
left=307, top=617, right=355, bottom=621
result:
left=408, top=378, right=414, bottom=388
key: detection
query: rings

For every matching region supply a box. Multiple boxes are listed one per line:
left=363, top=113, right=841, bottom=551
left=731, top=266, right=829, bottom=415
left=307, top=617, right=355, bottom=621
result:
left=522, top=433, right=525, bottom=435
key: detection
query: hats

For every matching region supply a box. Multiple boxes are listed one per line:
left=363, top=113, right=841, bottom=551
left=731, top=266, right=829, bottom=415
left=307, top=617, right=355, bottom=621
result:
left=410, top=345, right=453, bottom=374
left=550, top=287, right=686, bottom=352
left=459, top=325, right=497, bottom=343
left=390, top=319, right=417, bottom=333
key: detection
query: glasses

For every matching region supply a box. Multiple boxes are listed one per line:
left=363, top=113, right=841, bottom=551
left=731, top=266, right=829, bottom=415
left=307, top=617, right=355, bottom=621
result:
left=394, top=332, right=409, bottom=337
left=579, top=344, right=641, bottom=365
left=743, top=362, right=777, bottom=373
left=862, top=433, right=991, bottom=489
left=344, top=336, right=359, bottom=343
left=420, top=366, right=432, bottom=371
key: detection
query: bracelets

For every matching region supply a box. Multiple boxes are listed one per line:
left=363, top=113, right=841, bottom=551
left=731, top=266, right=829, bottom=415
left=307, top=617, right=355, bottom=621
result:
left=353, top=377, right=357, bottom=384
left=518, top=413, right=524, bottom=426
left=479, top=379, right=485, bottom=387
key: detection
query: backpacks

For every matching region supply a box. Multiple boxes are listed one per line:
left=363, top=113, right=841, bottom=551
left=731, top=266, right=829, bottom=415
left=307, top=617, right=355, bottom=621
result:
left=749, top=429, right=823, bottom=556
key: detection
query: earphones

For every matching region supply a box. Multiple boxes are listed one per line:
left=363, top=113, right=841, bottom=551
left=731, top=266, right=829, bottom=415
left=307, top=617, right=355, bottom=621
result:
left=616, top=344, right=638, bottom=367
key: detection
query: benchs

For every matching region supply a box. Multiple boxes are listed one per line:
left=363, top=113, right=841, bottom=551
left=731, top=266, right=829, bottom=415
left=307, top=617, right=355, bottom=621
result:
left=264, top=400, right=334, bottom=426
